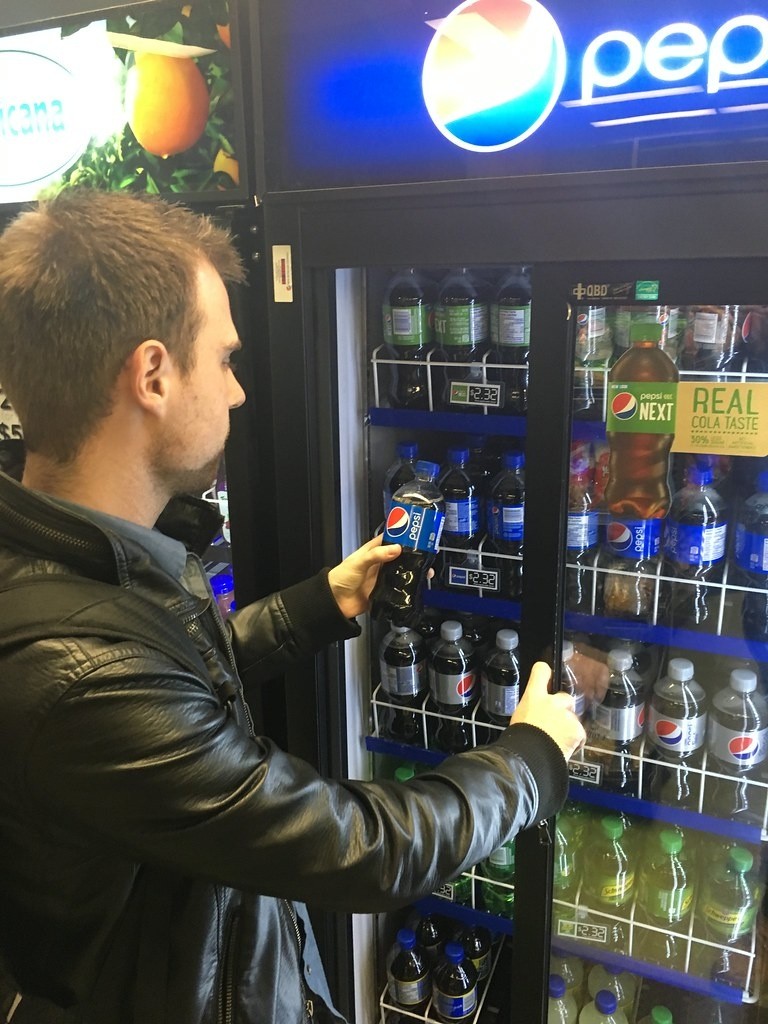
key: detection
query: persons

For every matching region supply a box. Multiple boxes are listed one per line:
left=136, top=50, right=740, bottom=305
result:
left=0, top=185, right=584, bottom=1024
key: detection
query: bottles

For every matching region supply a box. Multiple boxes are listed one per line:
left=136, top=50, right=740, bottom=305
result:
left=372, top=269, right=768, bottom=1024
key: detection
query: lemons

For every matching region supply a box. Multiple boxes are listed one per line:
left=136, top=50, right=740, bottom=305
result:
left=124, top=50, right=210, bottom=159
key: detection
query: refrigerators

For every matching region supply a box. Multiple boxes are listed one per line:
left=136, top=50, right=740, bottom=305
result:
left=241, top=3, right=768, bottom=1024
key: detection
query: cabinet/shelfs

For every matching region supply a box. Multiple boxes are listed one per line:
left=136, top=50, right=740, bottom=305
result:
left=365, top=352, right=767, bottom=1024
